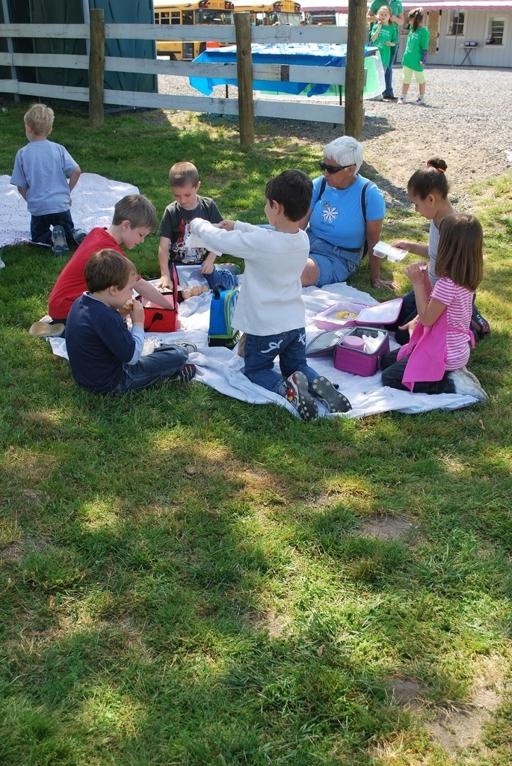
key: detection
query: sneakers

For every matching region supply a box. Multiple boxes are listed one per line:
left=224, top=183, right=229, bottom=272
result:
left=154, top=342, right=198, bottom=383
left=383, top=96, right=407, bottom=105
left=415, top=98, right=424, bottom=105
left=449, top=365, right=490, bottom=402
left=280, top=371, right=354, bottom=424
left=470, top=307, right=491, bottom=336
left=50, top=224, right=91, bottom=254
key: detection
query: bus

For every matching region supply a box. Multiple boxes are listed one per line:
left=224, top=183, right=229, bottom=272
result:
left=154, top=0, right=305, bottom=68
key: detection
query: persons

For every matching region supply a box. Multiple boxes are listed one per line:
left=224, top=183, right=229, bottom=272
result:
left=187, top=169, right=352, bottom=422
left=384, top=156, right=489, bottom=344
left=397, top=7, right=430, bottom=104
left=154, top=161, right=240, bottom=290
left=10, top=104, right=87, bottom=248
left=28, top=193, right=174, bottom=339
left=366, top=0, right=403, bottom=101
left=65, top=248, right=198, bottom=394
left=379, top=212, right=489, bottom=401
left=300, top=135, right=402, bottom=291
left=369, top=5, right=399, bottom=74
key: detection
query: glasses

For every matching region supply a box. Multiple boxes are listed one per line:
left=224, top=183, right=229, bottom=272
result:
left=318, top=161, right=358, bottom=174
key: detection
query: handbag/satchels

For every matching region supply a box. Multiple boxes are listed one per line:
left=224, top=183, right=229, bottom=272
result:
left=141, top=263, right=182, bottom=332
left=205, top=286, right=241, bottom=348
left=308, top=325, right=392, bottom=377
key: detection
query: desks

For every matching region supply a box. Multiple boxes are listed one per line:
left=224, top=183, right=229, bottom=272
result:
left=456, top=43, right=483, bottom=66
left=188, top=42, right=386, bottom=128
